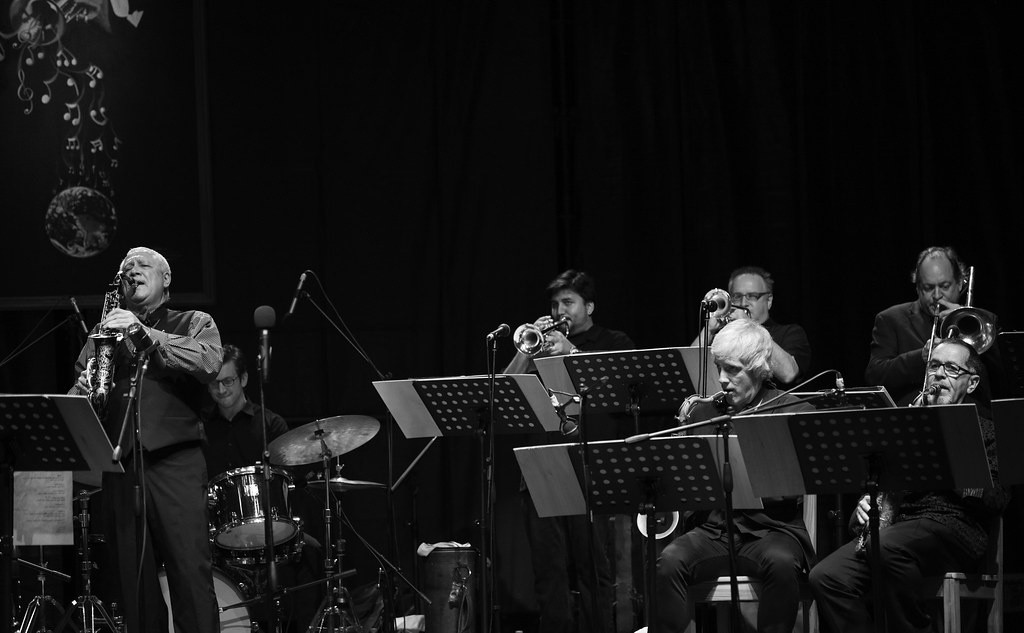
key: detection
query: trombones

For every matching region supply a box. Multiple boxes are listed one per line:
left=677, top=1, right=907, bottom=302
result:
left=923, top=264, right=997, bottom=392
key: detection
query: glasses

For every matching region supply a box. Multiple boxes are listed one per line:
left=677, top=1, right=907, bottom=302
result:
left=209, top=373, right=244, bottom=390
left=730, top=291, right=771, bottom=306
left=925, top=359, right=976, bottom=379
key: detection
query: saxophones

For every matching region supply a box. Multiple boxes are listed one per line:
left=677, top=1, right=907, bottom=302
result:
left=636, top=389, right=730, bottom=541
left=85, top=270, right=140, bottom=421
left=854, top=382, right=942, bottom=557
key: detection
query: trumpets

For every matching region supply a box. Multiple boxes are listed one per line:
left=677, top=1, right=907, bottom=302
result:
left=512, top=317, right=571, bottom=358
left=703, top=287, right=751, bottom=325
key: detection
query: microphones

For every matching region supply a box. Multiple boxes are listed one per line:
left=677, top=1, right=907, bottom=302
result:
left=70, top=298, right=89, bottom=333
left=836, top=372, right=848, bottom=407
left=945, top=325, right=960, bottom=339
left=700, top=300, right=718, bottom=312
left=289, top=273, right=306, bottom=314
left=547, top=389, right=569, bottom=422
left=126, top=323, right=166, bottom=374
left=254, top=305, right=276, bottom=382
left=487, top=323, right=510, bottom=341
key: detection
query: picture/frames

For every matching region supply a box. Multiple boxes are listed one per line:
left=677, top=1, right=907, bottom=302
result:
left=0, top=0, right=219, bottom=310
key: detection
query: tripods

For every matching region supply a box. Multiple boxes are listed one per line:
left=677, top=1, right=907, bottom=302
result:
left=306, top=435, right=364, bottom=633
left=0, top=394, right=125, bottom=633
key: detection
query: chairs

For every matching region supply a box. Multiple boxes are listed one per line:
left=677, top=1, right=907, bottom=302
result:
left=936, top=517, right=1004, bottom=633
left=695, top=495, right=819, bottom=633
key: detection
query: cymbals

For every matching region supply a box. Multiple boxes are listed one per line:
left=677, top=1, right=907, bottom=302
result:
left=305, top=477, right=385, bottom=488
left=267, top=414, right=382, bottom=467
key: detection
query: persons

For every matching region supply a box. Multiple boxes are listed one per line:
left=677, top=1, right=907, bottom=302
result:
left=691, top=268, right=811, bottom=384
left=68, top=247, right=224, bottom=633
left=808, top=340, right=1011, bottom=632
left=656, top=320, right=818, bottom=632
left=501, top=269, right=639, bottom=633
left=868, top=246, right=1020, bottom=392
left=200, top=345, right=289, bottom=466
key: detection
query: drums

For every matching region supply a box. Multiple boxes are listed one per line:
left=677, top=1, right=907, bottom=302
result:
left=206, top=465, right=299, bottom=552
left=156, top=543, right=296, bottom=633
